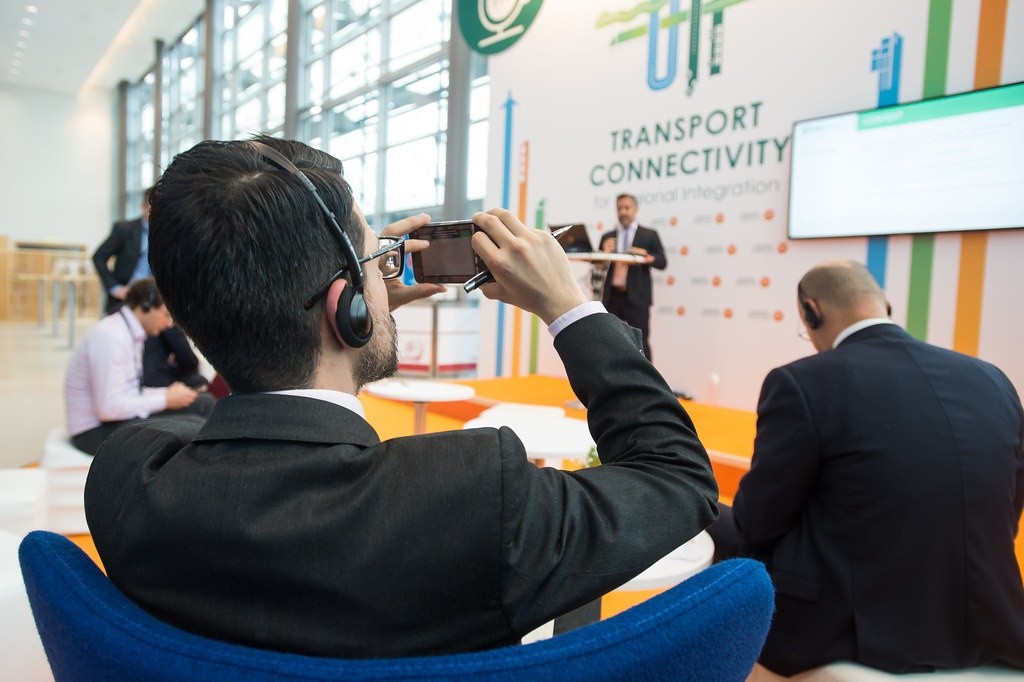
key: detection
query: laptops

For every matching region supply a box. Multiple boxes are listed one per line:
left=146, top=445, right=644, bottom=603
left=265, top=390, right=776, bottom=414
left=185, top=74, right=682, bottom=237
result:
left=548, top=225, right=605, bottom=253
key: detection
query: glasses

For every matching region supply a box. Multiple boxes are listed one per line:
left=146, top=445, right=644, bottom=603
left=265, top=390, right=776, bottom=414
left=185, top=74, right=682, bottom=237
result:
left=305, top=235, right=406, bottom=310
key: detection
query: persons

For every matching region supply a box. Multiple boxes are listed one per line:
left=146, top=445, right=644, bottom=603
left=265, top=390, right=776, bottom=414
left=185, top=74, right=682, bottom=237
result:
left=84, top=136, right=720, bottom=660
left=64, top=187, right=217, bottom=455
left=599, top=194, right=667, bottom=363
left=707, top=263, right=1023, bottom=677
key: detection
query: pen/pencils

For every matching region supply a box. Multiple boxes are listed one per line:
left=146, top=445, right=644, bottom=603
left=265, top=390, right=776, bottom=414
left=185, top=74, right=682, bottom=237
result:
left=463, top=223, right=573, bottom=293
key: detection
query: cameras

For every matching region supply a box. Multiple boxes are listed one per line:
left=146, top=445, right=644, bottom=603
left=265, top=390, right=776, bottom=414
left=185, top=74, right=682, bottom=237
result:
left=408, top=220, right=495, bottom=285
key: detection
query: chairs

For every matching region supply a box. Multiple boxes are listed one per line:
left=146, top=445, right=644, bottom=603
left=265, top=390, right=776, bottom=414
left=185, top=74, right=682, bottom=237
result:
left=17, top=529, right=774, bottom=682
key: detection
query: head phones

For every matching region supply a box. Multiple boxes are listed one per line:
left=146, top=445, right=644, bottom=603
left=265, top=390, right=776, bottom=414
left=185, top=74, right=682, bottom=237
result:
left=797, top=281, right=892, bottom=330
left=139, top=278, right=158, bottom=312
left=236, top=139, right=375, bottom=348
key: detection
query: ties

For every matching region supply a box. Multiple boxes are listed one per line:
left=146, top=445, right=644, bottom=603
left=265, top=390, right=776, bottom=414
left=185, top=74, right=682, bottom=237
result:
left=624, top=228, right=627, bottom=252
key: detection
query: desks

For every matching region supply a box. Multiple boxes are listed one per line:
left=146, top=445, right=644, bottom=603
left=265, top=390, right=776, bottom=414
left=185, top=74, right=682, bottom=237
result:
left=464, top=417, right=596, bottom=469
left=552, top=527, right=715, bottom=637
left=365, top=379, right=478, bottom=435
